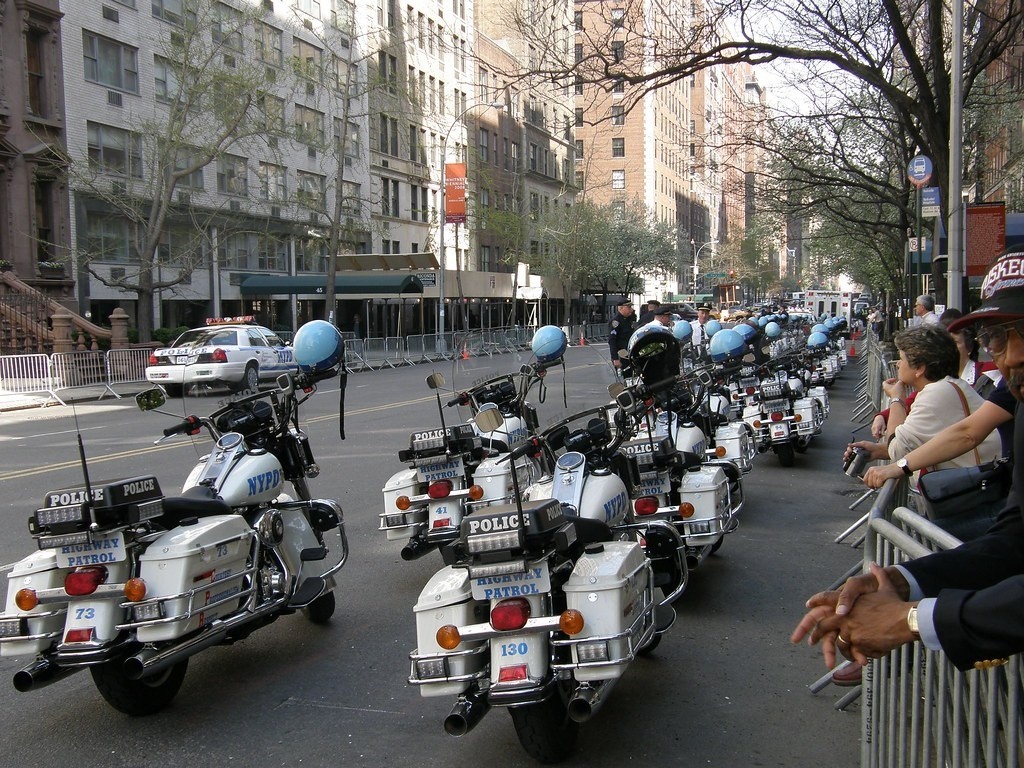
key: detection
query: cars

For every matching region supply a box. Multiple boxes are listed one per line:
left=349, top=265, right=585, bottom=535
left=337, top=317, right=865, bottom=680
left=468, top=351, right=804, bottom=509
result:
left=146, top=315, right=299, bottom=397
left=851, top=292, right=872, bottom=332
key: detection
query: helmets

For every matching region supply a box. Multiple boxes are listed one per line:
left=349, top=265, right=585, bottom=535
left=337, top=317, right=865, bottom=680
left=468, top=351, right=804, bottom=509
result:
left=709, top=329, right=744, bottom=361
left=748, top=313, right=847, bottom=336
left=532, top=325, right=567, bottom=361
left=627, top=324, right=681, bottom=385
left=674, top=320, right=693, bottom=341
left=806, top=332, right=828, bottom=350
left=732, top=324, right=755, bottom=340
left=706, top=319, right=723, bottom=337
left=293, top=320, right=344, bottom=373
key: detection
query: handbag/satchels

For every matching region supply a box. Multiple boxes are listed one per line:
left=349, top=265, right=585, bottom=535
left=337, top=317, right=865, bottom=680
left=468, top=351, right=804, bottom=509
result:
left=919, top=450, right=1014, bottom=543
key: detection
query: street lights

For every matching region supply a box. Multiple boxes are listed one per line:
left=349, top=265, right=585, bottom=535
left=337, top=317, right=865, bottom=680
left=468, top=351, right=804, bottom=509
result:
left=435, top=102, right=506, bottom=355
left=694, top=240, right=720, bottom=310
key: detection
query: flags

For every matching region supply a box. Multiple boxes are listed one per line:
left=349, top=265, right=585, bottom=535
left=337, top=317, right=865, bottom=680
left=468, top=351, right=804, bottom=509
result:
left=446, top=163, right=465, bottom=223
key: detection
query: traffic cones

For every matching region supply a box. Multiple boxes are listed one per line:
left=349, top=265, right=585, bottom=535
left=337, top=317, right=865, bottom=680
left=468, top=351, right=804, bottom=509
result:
left=857, top=327, right=862, bottom=338
left=851, top=327, right=858, bottom=340
left=848, top=336, right=858, bottom=357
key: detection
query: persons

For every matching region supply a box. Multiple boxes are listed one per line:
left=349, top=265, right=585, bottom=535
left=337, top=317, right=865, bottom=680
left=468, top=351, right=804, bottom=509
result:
left=689, top=302, right=714, bottom=356
left=609, top=299, right=636, bottom=387
left=777, top=306, right=788, bottom=319
left=790, top=243, right=1024, bottom=688
left=762, top=306, right=774, bottom=316
left=633, top=300, right=673, bottom=338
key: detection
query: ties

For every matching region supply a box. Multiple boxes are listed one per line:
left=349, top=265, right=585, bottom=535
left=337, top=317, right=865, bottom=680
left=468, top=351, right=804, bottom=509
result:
left=700, top=325, right=707, bottom=357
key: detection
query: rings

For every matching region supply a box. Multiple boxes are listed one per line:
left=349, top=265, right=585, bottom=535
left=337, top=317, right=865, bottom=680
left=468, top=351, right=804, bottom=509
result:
left=838, top=636, right=850, bottom=644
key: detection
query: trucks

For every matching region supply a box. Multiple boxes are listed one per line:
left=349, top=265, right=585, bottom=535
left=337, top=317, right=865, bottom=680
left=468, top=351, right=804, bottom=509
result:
left=804, top=290, right=860, bottom=340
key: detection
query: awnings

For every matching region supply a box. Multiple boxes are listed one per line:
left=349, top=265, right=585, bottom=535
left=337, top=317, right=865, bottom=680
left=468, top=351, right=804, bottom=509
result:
left=905, top=212, right=1024, bottom=296
left=240, top=274, right=423, bottom=336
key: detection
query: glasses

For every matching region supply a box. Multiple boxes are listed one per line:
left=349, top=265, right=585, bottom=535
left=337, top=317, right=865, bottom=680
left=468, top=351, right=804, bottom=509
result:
left=974, top=318, right=1024, bottom=357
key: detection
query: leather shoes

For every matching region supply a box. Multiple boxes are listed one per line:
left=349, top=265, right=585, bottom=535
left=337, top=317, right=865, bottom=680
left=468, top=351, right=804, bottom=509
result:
left=831, top=659, right=913, bottom=687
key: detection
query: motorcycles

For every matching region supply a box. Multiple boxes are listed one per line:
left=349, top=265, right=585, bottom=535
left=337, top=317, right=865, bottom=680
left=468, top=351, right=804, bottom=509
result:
left=376, top=310, right=849, bottom=764
left=0, top=358, right=357, bottom=721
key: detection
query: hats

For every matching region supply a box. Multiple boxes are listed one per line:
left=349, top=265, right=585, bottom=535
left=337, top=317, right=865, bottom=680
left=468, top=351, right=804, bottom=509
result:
left=697, top=302, right=712, bottom=311
left=947, top=243, right=1024, bottom=333
left=647, top=300, right=660, bottom=305
left=616, top=298, right=634, bottom=306
left=654, top=307, right=673, bottom=315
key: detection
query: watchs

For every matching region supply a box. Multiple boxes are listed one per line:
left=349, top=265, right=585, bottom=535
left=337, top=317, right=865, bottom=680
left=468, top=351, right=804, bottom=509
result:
left=890, top=397, right=908, bottom=409
left=898, top=457, right=914, bottom=476
left=908, top=605, right=922, bottom=642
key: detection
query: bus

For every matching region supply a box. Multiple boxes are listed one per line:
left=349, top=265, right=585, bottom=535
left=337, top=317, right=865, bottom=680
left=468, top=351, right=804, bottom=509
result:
left=663, top=293, right=714, bottom=307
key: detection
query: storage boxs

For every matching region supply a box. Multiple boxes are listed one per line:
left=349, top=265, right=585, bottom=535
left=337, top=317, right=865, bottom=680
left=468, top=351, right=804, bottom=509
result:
left=381, top=337, right=847, bottom=699
left=0, top=515, right=256, bottom=657
